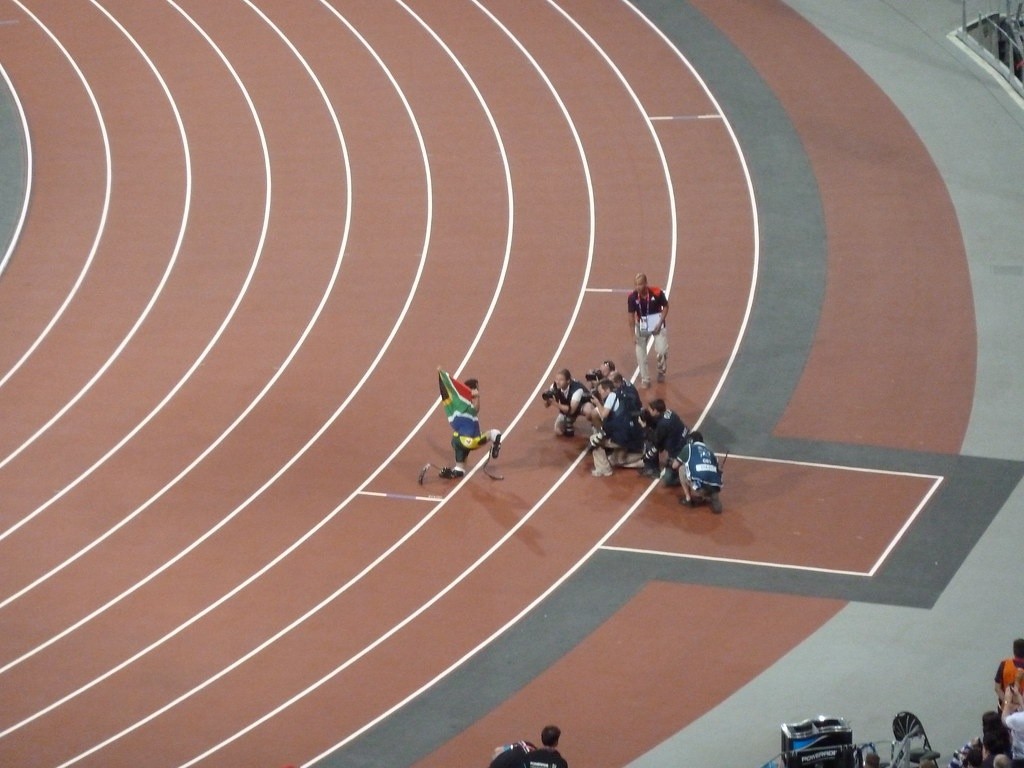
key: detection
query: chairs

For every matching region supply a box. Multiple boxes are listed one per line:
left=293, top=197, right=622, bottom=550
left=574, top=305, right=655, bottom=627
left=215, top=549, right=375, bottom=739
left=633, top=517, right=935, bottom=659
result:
left=892, top=711, right=940, bottom=768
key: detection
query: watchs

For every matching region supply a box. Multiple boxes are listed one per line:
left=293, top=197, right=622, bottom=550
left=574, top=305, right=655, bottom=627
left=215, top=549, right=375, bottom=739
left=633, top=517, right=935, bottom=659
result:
left=1004, top=700, right=1011, bottom=704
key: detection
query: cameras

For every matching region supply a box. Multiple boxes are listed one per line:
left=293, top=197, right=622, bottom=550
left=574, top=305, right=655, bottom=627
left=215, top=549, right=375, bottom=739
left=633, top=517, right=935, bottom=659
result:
left=542, top=388, right=563, bottom=402
left=631, top=408, right=651, bottom=422
left=582, top=391, right=601, bottom=402
left=586, top=368, right=602, bottom=381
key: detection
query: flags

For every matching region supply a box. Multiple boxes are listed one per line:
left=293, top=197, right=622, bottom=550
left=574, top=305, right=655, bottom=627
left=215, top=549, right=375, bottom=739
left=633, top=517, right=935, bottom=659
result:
left=437, top=369, right=482, bottom=437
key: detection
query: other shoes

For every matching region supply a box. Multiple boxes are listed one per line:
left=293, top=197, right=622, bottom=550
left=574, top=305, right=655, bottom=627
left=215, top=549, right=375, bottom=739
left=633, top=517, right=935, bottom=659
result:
left=639, top=469, right=660, bottom=478
left=680, top=497, right=694, bottom=507
left=642, top=378, right=650, bottom=388
left=657, top=372, right=665, bottom=382
left=591, top=468, right=612, bottom=476
left=710, top=491, right=723, bottom=513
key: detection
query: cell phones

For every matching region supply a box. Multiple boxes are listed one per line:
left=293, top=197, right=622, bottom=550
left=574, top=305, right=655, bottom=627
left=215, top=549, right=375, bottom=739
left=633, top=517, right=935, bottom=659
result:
left=1009, top=683, right=1015, bottom=692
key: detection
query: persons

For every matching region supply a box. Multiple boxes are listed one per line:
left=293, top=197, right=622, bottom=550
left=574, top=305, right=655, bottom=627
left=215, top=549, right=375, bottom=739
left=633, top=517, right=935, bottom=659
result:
left=946, top=639, right=1024, bottom=768
left=672, top=431, right=723, bottom=513
left=865, top=752, right=880, bottom=768
left=522, top=723, right=569, bottom=768
left=418, top=365, right=504, bottom=485
left=628, top=272, right=669, bottom=392
left=919, top=759, right=937, bottom=768
left=542, top=360, right=692, bottom=489
